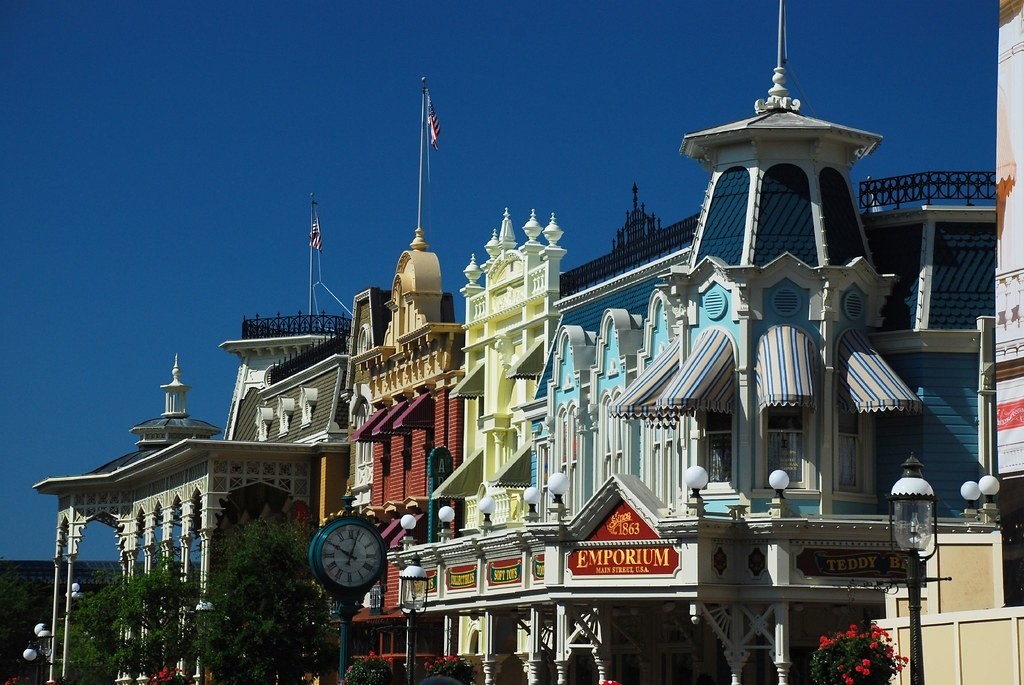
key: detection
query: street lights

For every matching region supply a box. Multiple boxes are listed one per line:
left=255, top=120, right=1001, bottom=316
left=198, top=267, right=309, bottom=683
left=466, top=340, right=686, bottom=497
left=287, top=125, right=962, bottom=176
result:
left=22, top=622, right=52, bottom=685
left=874, top=451, right=954, bottom=685
left=196, top=597, right=215, bottom=685
left=395, top=551, right=432, bottom=685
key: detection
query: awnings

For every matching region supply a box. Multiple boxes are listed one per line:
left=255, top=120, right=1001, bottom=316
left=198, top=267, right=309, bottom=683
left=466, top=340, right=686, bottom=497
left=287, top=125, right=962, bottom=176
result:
left=607, top=329, right=817, bottom=431
left=375, top=513, right=428, bottom=550
left=351, top=392, right=434, bottom=444
left=838, top=329, right=923, bottom=416
left=449, top=338, right=544, bottom=400
left=431, top=439, right=532, bottom=499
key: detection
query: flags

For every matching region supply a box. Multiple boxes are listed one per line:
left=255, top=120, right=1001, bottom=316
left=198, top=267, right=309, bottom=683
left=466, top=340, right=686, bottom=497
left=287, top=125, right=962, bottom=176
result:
left=309, top=202, right=323, bottom=254
left=420, top=89, right=441, bottom=152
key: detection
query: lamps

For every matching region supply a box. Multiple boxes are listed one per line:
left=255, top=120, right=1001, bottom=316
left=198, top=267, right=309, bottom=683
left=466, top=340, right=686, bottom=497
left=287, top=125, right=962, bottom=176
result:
left=548, top=473, right=569, bottom=504
left=478, top=497, right=494, bottom=523
left=438, top=506, right=456, bottom=530
left=980, top=475, right=999, bottom=503
left=960, top=480, right=982, bottom=510
left=684, top=465, right=710, bottom=498
left=523, top=487, right=541, bottom=512
left=400, top=515, right=416, bottom=537
left=769, top=469, right=789, bottom=499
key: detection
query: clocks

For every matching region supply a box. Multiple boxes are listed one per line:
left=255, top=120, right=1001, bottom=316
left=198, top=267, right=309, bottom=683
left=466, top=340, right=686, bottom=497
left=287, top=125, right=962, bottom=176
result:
left=317, top=522, right=388, bottom=590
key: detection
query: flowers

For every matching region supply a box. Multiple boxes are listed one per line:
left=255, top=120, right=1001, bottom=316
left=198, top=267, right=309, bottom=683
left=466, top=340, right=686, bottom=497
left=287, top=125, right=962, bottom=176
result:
left=808, top=621, right=910, bottom=685
left=5, top=677, right=35, bottom=685
left=424, top=653, right=478, bottom=685
left=150, top=667, right=196, bottom=685
left=338, top=650, right=395, bottom=685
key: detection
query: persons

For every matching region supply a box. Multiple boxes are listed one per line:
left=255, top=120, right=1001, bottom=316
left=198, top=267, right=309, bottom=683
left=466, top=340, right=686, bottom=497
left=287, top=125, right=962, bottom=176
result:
left=417, top=676, right=464, bottom=685
